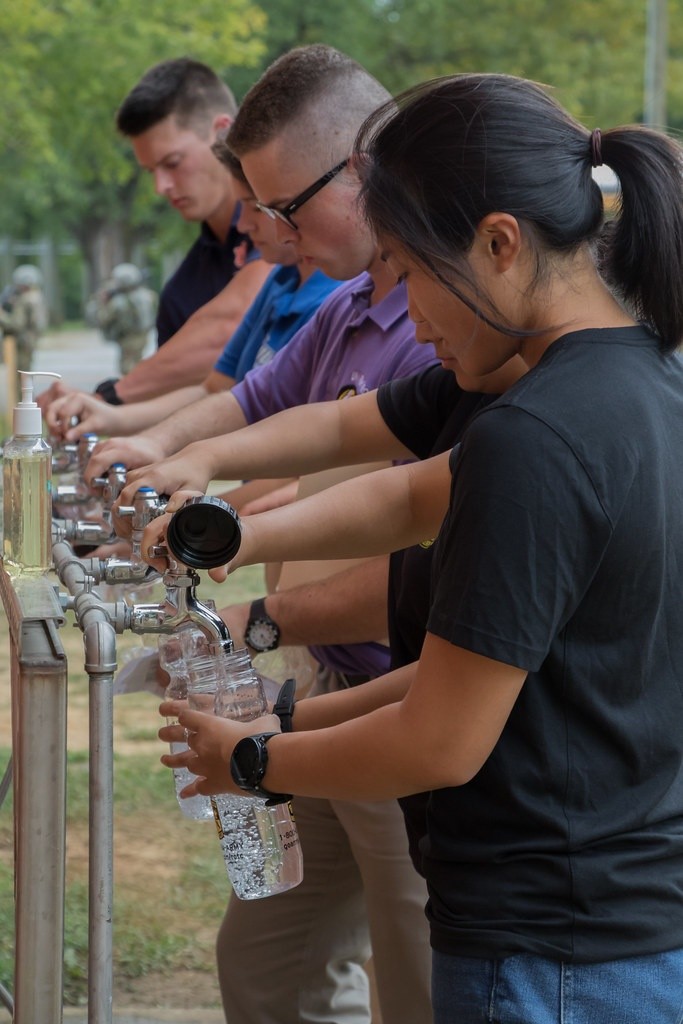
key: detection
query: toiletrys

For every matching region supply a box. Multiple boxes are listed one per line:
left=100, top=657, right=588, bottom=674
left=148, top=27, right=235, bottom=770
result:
left=1, top=370, right=64, bottom=573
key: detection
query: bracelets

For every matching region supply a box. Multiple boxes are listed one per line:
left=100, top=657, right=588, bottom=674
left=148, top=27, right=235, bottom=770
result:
left=96, top=378, right=122, bottom=405
left=272, top=678, right=298, bottom=733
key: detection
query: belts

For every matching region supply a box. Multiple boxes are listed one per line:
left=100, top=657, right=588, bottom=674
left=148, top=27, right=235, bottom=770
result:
left=334, top=672, right=370, bottom=692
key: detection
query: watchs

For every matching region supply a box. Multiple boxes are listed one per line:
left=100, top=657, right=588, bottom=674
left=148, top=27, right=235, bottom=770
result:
left=243, top=596, right=281, bottom=653
left=230, top=732, right=293, bottom=806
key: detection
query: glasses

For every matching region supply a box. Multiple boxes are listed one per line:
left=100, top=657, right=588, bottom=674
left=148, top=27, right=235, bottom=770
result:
left=254, top=159, right=352, bottom=232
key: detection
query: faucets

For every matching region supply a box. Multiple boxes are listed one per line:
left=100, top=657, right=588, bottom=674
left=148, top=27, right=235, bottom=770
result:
left=58, top=431, right=98, bottom=505
left=105, top=487, right=174, bottom=587
left=78, top=461, right=129, bottom=548
left=53, top=414, right=79, bottom=473
left=131, top=540, right=236, bottom=662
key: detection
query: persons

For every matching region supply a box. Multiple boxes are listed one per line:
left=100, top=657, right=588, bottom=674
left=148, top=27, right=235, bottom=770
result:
left=0, top=263, right=45, bottom=402
left=97, top=264, right=158, bottom=375
left=37, top=43, right=682, bottom=1024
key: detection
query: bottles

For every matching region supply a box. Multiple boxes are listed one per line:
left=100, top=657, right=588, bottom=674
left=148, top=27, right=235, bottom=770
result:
left=106, top=564, right=222, bottom=821
left=187, top=650, right=303, bottom=899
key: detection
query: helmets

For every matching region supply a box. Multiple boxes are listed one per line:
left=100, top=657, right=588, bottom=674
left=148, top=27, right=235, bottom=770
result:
left=112, top=262, right=142, bottom=290
left=12, top=265, right=43, bottom=288
